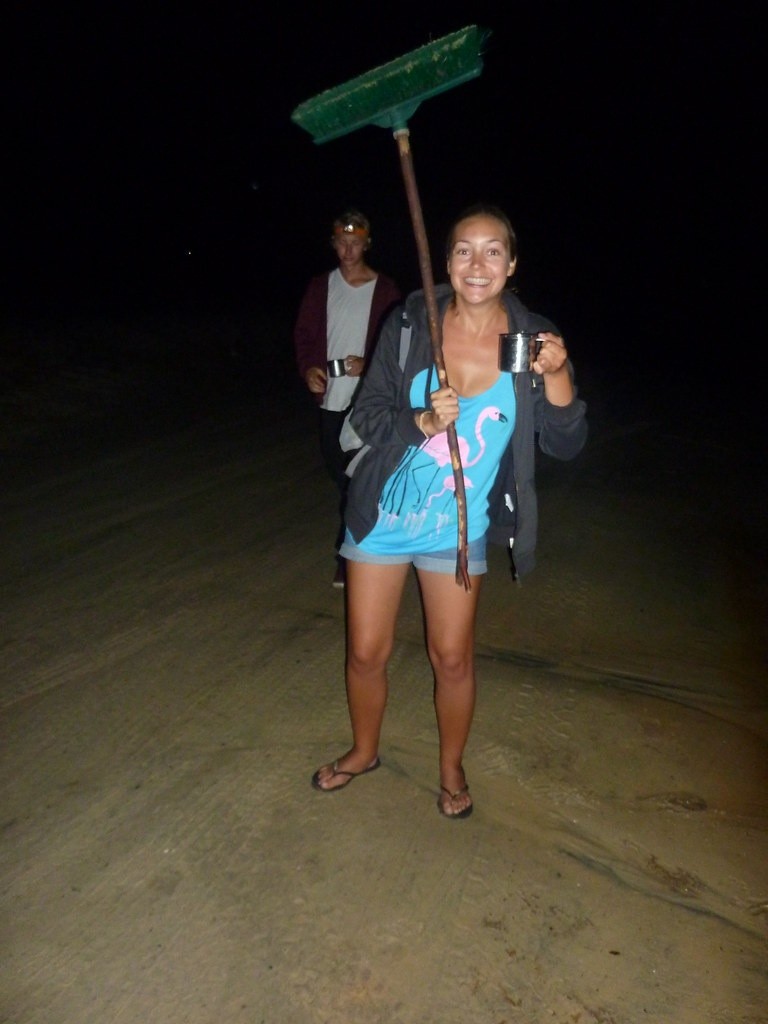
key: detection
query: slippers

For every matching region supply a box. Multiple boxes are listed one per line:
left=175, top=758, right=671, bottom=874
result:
left=437, top=782, right=473, bottom=819
left=312, top=757, right=380, bottom=792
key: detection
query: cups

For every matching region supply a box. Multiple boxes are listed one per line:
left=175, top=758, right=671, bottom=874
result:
left=327, top=358, right=354, bottom=378
left=497, top=333, right=548, bottom=373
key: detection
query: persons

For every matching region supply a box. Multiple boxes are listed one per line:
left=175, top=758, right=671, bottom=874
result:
left=298, top=216, right=399, bottom=591
left=308, top=209, right=589, bottom=817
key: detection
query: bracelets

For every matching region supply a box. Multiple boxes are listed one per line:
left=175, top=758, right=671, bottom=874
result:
left=420, top=408, right=433, bottom=438
